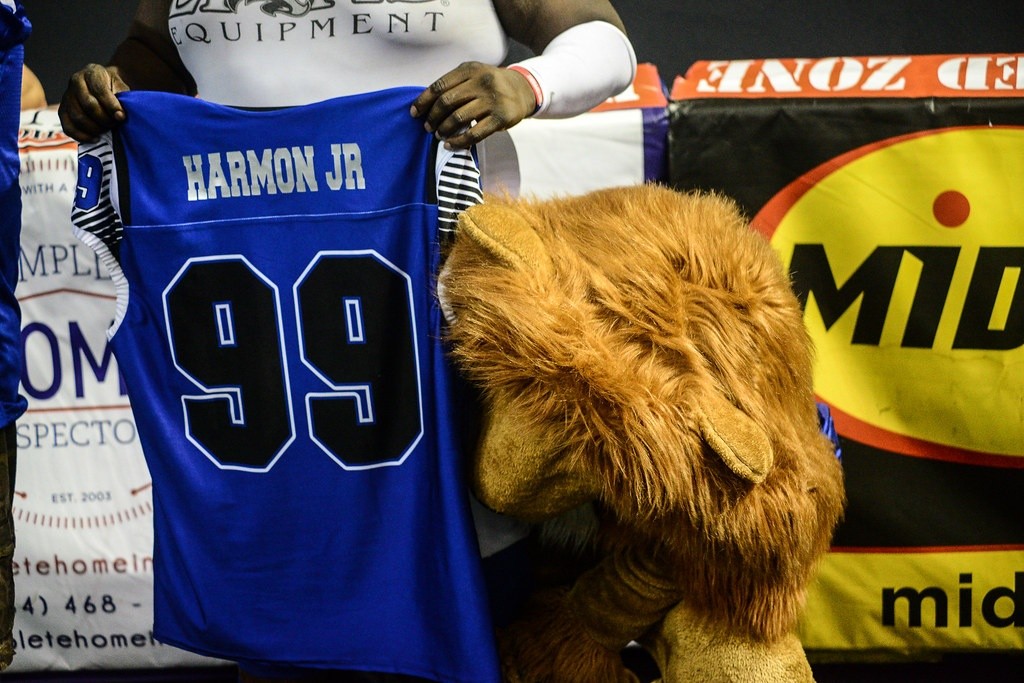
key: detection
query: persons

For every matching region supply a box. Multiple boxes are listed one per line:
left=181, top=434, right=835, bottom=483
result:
left=58, top=0, right=638, bottom=683
left=0, top=0, right=33, bottom=671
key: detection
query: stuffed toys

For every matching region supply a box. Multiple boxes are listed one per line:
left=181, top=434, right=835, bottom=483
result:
left=438, top=184, right=846, bottom=683
